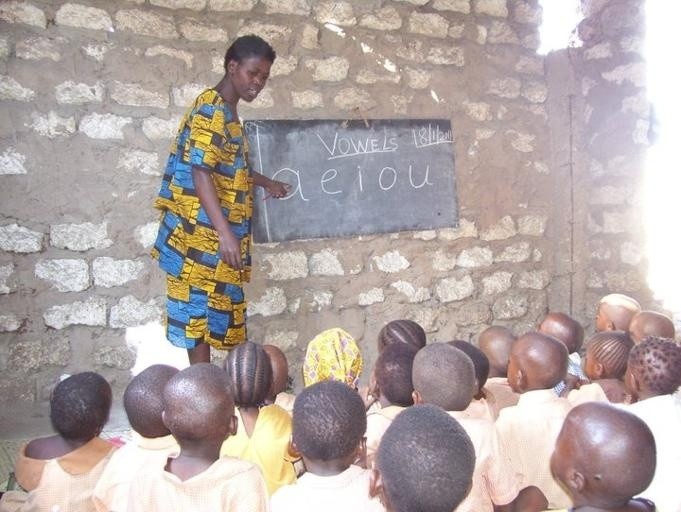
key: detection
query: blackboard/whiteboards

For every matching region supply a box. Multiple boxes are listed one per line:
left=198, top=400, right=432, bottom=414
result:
left=242, top=119, right=458, bottom=243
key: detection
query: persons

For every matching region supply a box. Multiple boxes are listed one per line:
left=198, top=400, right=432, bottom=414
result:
left=0, top=292, right=681, bottom=512
left=151, top=35, right=292, bottom=365
